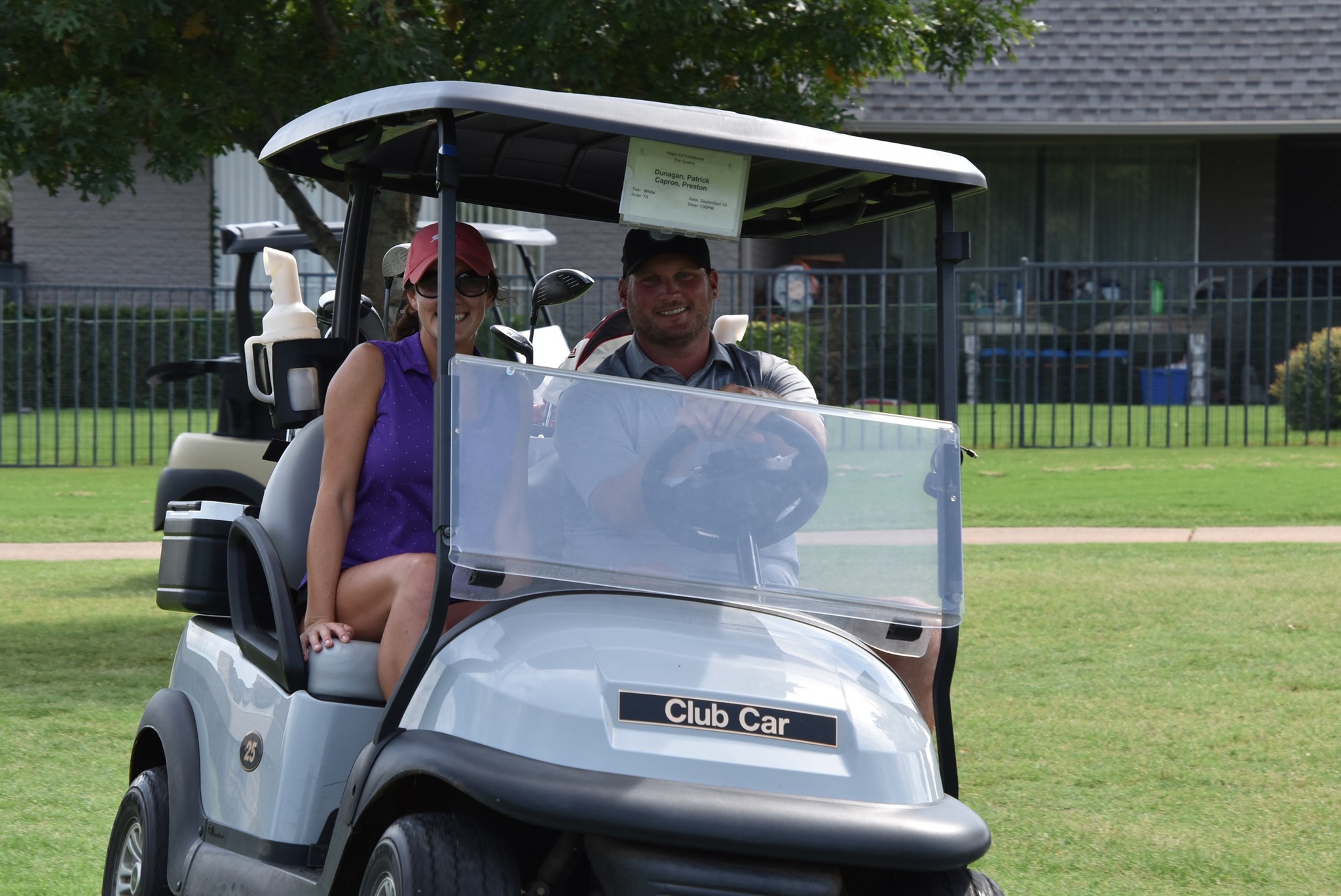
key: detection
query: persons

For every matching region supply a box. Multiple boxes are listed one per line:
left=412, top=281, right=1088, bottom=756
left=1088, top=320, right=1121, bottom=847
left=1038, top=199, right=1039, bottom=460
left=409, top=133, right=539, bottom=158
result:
left=551, top=226, right=942, bottom=743
left=294, top=222, right=535, bottom=722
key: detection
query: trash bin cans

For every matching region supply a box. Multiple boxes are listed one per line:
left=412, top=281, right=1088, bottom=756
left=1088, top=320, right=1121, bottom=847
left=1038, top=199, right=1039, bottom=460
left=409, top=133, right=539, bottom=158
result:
left=1140, top=366, right=1188, bottom=405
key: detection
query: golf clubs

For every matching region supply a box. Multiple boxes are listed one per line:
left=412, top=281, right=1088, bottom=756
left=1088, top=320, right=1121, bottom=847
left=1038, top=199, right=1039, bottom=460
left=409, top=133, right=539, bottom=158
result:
left=383, top=243, right=411, bottom=342
left=526, top=268, right=595, bottom=367
left=489, top=324, right=533, bottom=365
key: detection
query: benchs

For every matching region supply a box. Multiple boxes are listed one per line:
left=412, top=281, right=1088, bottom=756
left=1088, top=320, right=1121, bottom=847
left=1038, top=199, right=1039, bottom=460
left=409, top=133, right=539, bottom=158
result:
left=226, top=415, right=801, bottom=700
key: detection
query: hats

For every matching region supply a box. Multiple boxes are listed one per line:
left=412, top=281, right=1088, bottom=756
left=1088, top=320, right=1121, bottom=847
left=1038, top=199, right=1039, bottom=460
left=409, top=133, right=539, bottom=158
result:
left=621, top=227, right=711, bottom=278
left=403, top=220, right=495, bottom=290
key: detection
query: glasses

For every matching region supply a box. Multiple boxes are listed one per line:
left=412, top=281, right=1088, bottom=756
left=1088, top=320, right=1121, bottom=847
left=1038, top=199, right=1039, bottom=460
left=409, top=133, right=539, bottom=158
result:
left=415, top=268, right=492, bottom=299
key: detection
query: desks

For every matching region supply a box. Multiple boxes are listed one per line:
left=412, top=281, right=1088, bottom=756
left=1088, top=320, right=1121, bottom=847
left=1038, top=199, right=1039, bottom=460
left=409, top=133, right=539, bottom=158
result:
left=961, top=311, right=1208, bottom=404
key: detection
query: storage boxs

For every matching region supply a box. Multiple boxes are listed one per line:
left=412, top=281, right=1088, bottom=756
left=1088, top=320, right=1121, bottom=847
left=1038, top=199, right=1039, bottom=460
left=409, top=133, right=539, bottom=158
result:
left=1139, top=362, right=1188, bottom=404
left=155, top=500, right=259, bottom=616
